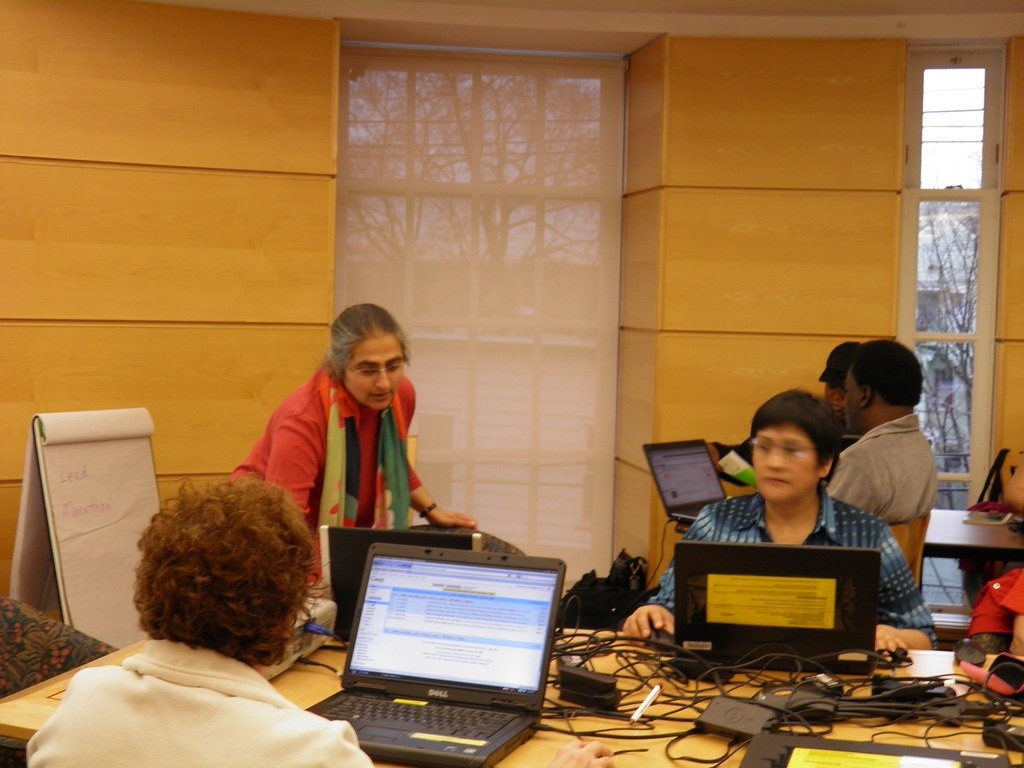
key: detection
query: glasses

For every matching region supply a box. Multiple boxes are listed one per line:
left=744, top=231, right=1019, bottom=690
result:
left=749, top=435, right=817, bottom=464
left=345, top=361, right=409, bottom=380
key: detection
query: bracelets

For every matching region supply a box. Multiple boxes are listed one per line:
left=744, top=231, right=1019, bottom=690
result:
left=419, top=503, right=437, bottom=518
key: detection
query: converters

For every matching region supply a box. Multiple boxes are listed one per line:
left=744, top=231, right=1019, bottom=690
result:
left=557, top=664, right=619, bottom=712
left=694, top=698, right=780, bottom=743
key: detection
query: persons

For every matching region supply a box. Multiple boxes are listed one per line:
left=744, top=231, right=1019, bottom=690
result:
left=623, top=389, right=937, bottom=662
left=706, top=341, right=862, bottom=487
left=967, top=568, right=1024, bottom=656
left=25, top=472, right=614, bottom=767
left=826, top=339, right=937, bottom=523
left=227, top=304, right=477, bottom=601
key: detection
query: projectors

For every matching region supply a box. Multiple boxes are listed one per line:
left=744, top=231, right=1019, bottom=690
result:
left=248, top=596, right=335, bottom=681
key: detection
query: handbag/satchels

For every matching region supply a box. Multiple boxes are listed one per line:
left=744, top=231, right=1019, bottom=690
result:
left=562, top=547, right=651, bottom=630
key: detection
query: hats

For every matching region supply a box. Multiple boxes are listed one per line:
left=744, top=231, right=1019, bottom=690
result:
left=818, top=342, right=862, bottom=386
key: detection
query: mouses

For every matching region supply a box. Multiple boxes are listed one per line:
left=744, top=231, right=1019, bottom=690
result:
left=955, top=637, right=987, bottom=667
left=752, top=686, right=838, bottom=718
left=644, top=624, right=674, bottom=653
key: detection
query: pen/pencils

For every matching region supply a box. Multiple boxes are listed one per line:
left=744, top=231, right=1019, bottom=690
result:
left=625, top=681, right=664, bottom=728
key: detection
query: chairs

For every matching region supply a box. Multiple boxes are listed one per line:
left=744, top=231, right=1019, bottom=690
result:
left=886, top=510, right=931, bottom=587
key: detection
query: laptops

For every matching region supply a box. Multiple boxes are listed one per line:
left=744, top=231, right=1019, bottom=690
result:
left=320, top=525, right=483, bottom=642
left=643, top=438, right=727, bottom=526
left=672, top=541, right=881, bottom=675
left=739, top=730, right=1012, bottom=768
left=302, top=543, right=567, bottom=768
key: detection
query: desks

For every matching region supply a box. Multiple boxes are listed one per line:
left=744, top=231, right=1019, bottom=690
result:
left=919, top=508, right=1024, bottom=594
left=0, top=630, right=1024, bottom=768
